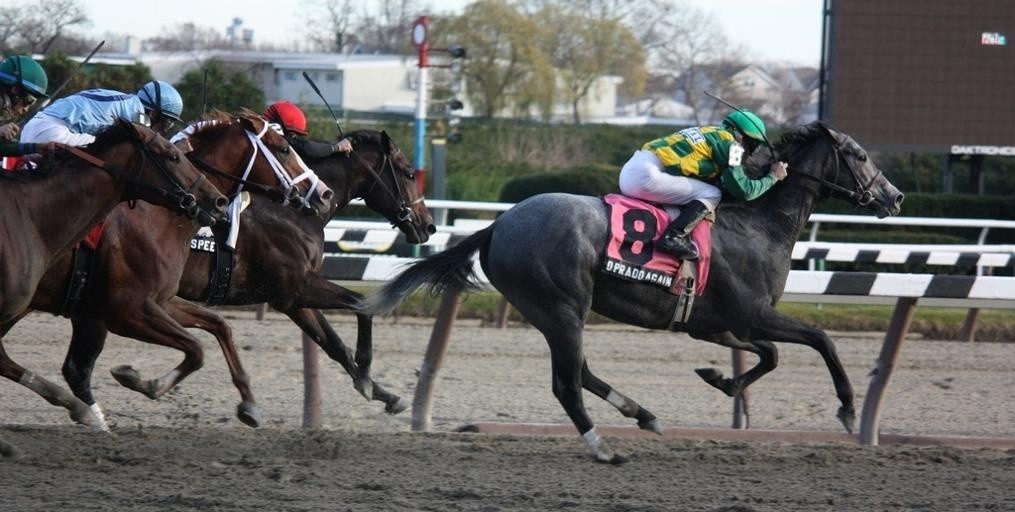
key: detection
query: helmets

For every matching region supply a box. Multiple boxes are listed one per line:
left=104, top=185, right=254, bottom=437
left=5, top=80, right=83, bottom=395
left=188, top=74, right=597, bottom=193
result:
left=263, top=97, right=308, bottom=137
left=0, top=52, right=49, bottom=101
left=724, top=110, right=769, bottom=146
left=137, top=78, right=186, bottom=124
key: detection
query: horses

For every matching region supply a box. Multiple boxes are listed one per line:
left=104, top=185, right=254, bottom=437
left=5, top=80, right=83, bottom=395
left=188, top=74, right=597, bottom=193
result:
left=335, top=118, right=907, bottom=465
left=28, top=108, right=334, bottom=429
left=170, top=103, right=436, bottom=418
left=2, top=115, right=234, bottom=434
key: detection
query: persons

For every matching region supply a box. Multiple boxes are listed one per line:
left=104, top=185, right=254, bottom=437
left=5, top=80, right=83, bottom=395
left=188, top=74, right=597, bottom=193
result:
left=617, top=108, right=789, bottom=262
left=168, top=98, right=355, bottom=161
left=14, top=79, right=186, bottom=158
left=0, top=54, right=49, bottom=145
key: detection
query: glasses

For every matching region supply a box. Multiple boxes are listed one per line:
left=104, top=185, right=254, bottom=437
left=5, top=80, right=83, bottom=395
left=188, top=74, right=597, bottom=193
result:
left=18, top=94, right=36, bottom=105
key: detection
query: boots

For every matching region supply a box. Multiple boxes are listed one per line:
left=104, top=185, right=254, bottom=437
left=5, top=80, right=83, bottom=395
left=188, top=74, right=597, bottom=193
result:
left=653, top=198, right=711, bottom=260
